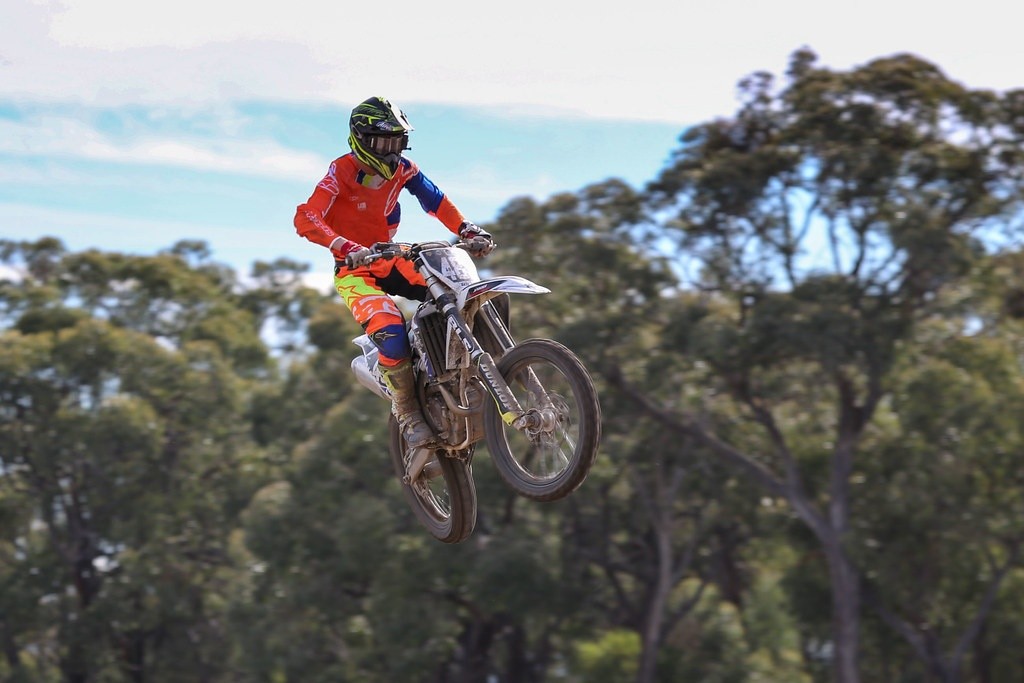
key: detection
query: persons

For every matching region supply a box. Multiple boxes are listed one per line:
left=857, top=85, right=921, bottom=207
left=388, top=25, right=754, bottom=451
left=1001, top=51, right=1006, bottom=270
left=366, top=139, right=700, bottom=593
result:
left=295, top=96, right=494, bottom=448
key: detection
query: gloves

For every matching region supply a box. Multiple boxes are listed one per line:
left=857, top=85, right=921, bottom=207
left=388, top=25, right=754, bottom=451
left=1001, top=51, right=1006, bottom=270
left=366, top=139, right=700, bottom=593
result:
left=331, top=237, right=371, bottom=270
left=458, top=219, right=493, bottom=259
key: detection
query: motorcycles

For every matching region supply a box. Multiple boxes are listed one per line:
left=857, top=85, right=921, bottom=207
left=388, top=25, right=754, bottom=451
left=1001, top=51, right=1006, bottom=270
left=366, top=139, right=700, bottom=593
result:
left=345, top=238, right=603, bottom=543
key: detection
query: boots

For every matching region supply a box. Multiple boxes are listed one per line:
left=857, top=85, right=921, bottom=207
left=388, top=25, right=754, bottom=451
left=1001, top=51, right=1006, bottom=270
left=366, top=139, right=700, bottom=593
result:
left=378, top=357, right=435, bottom=448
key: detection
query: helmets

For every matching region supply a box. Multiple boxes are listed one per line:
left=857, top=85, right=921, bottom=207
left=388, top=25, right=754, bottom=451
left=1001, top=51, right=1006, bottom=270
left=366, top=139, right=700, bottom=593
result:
left=347, top=97, right=414, bottom=182
